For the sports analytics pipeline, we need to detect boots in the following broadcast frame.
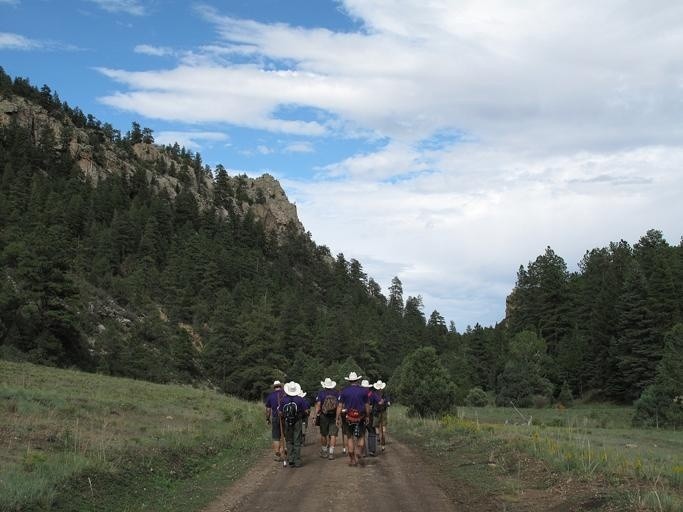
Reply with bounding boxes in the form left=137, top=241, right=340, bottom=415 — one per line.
left=355, top=445, right=367, bottom=467
left=347, top=451, right=358, bottom=466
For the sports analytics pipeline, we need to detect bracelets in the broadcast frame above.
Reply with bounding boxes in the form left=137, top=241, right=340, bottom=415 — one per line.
left=365, top=415, right=369, bottom=417
left=335, top=418, right=340, bottom=421
left=311, top=417, right=316, bottom=420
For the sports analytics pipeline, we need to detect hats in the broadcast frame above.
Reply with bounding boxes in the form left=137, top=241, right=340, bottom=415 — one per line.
left=270, top=379, right=284, bottom=390
left=373, top=380, right=387, bottom=390
left=359, top=379, right=373, bottom=388
left=297, top=390, right=307, bottom=398
left=344, top=371, right=363, bottom=382
left=283, top=381, right=301, bottom=397
left=320, top=377, right=337, bottom=389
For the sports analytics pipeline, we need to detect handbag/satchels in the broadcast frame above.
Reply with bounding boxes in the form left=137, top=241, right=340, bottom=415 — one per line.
left=345, top=406, right=363, bottom=425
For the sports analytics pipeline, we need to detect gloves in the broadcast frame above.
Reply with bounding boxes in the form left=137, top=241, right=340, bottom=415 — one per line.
left=265, top=417, right=272, bottom=427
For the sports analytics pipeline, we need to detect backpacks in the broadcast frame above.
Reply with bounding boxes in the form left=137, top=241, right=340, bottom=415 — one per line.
left=282, top=401, right=299, bottom=427
left=322, top=390, right=338, bottom=416
left=374, top=393, right=387, bottom=412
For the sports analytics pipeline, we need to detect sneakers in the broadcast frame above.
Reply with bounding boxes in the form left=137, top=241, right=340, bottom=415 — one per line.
left=319, top=450, right=328, bottom=458
left=365, top=440, right=386, bottom=457
left=327, top=452, right=336, bottom=460
left=272, top=442, right=306, bottom=470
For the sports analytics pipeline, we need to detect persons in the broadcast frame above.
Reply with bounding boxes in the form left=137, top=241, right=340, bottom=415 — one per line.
left=311, top=377, right=341, bottom=462
left=277, top=381, right=308, bottom=469
left=359, top=379, right=385, bottom=458
left=334, top=371, right=370, bottom=468
left=372, top=379, right=390, bottom=444
left=264, top=378, right=287, bottom=463
left=296, top=389, right=312, bottom=447
